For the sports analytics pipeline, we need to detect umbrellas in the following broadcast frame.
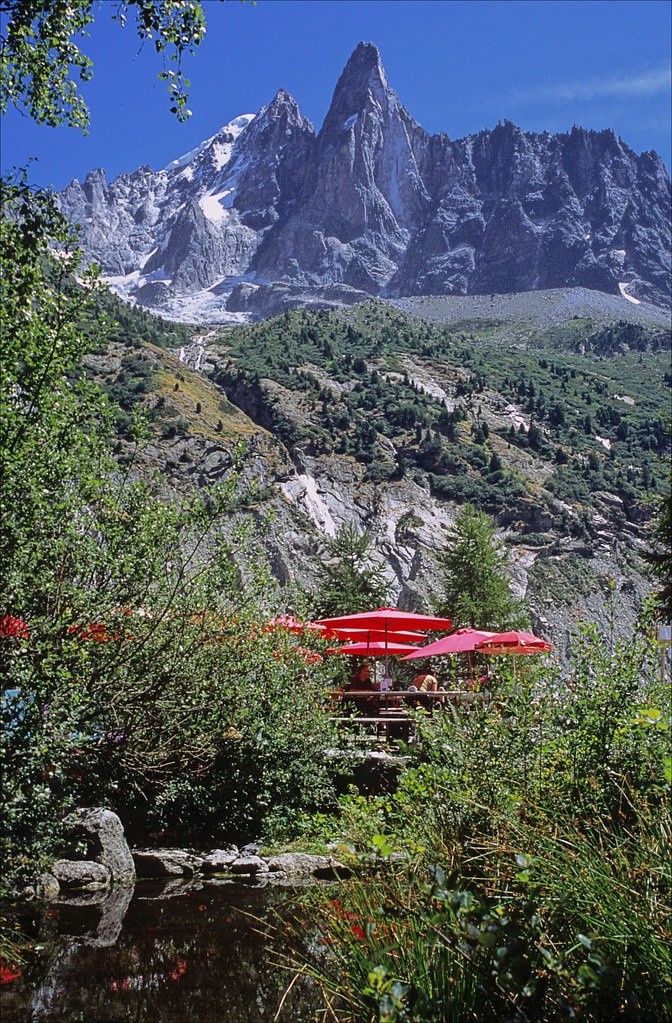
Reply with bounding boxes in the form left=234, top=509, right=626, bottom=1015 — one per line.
left=474, top=630, right=552, bottom=711
left=227, top=613, right=327, bottom=658
left=271, top=640, right=324, bottom=665
left=316, top=607, right=453, bottom=712
left=326, top=642, right=423, bottom=686
left=400, top=628, right=501, bottom=691
left=313, top=626, right=425, bottom=666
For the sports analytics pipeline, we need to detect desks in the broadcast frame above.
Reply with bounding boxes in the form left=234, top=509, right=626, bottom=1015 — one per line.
left=329, top=690, right=468, bottom=697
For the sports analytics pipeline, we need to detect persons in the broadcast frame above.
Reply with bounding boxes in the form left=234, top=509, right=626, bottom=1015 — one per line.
left=349, top=664, right=386, bottom=734
left=404, top=670, right=438, bottom=718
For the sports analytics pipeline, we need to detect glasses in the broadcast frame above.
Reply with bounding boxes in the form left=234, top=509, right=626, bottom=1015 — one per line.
left=364, top=670, right=369, bottom=672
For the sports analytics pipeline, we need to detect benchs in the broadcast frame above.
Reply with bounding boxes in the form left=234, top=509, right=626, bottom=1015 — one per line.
left=327, top=706, right=463, bottom=743
